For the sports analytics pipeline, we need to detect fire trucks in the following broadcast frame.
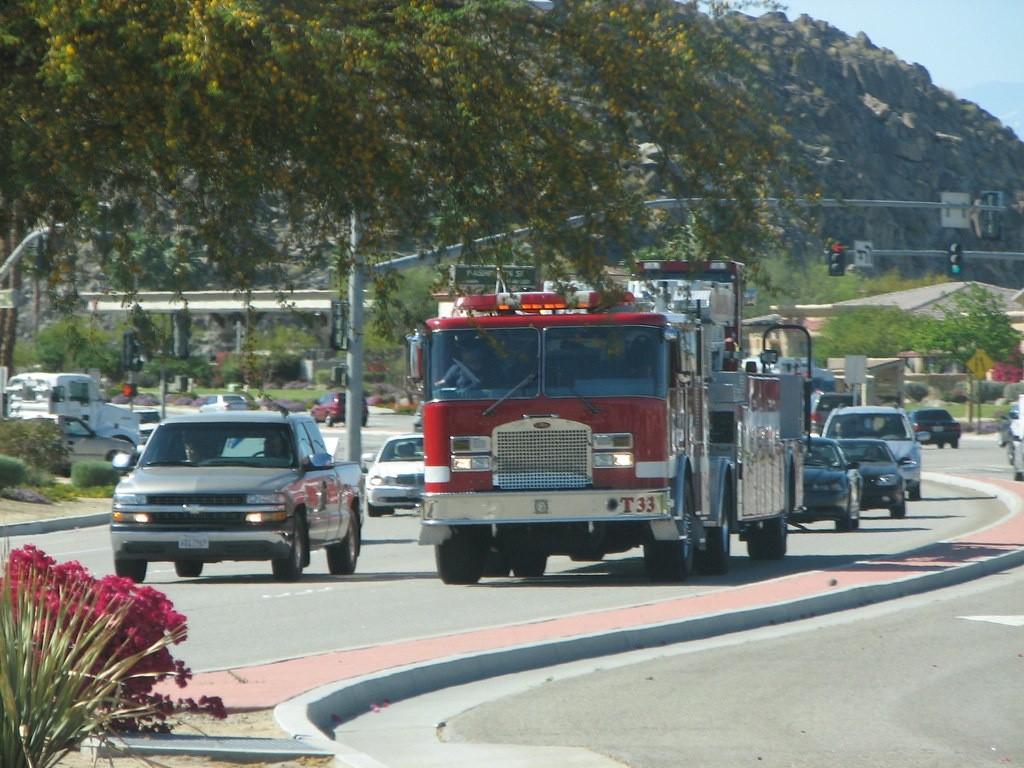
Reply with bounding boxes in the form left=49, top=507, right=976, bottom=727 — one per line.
left=406, top=259, right=813, bottom=586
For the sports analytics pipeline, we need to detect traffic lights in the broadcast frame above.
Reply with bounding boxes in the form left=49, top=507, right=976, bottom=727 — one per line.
left=945, top=239, right=964, bottom=280
left=123, top=384, right=139, bottom=398
left=829, top=240, right=845, bottom=278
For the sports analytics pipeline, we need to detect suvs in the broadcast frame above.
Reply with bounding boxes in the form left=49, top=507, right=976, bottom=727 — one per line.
left=809, top=389, right=862, bottom=439
left=822, top=402, right=930, bottom=504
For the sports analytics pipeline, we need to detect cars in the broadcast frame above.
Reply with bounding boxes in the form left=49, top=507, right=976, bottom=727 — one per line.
left=23, top=412, right=136, bottom=469
left=833, top=438, right=913, bottom=519
left=362, top=433, right=426, bottom=517
left=309, top=390, right=370, bottom=428
left=413, top=400, right=429, bottom=431
left=906, top=409, right=962, bottom=449
left=802, top=436, right=862, bottom=533
left=1000, top=393, right=1024, bottom=482
left=199, top=392, right=255, bottom=410
left=138, top=407, right=163, bottom=439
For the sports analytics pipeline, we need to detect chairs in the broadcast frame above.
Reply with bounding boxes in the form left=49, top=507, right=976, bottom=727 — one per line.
left=265, top=438, right=288, bottom=458
left=398, top=445, right=407, bottom=456
left=864, top=447, right=883, bottom=458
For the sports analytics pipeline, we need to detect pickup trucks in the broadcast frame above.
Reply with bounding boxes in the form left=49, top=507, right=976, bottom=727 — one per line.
left=110, top=410, right=365, bottom=584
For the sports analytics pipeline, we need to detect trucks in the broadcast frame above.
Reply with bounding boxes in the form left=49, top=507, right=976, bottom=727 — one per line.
left=8, top=370, right=142, bottom=451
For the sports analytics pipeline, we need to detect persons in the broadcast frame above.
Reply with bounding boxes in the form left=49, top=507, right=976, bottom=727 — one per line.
left=263, top=432, right=287, bottom=459
left=179, top=433, right=208, bottom=463
left=431, top=339, right=512, bottom=391
left=555, top=332, right=653, bottom=386
left=810, top=446, right=833, bottom=466
left=865, top=445, right=883, bottom=463
left=834, top=420, right=849, bottom=440
left=879, top=420, right=898, bottom=435
